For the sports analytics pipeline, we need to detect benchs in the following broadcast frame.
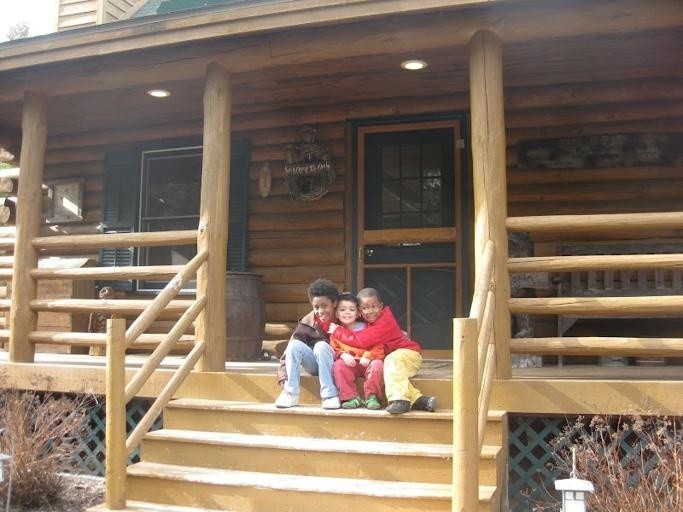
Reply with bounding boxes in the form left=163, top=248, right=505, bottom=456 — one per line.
left=550, top=236, right=683, bottom=369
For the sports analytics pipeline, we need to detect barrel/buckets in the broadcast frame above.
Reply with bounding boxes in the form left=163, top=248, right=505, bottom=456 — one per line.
left=226, top=269, right=266, bottom=360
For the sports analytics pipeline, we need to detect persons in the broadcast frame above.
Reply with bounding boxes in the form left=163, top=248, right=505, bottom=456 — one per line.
left=275, top=279, right=436, bottom=414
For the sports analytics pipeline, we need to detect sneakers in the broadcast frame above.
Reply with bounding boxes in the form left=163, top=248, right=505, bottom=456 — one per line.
left=415, top=396, right=436, bottom=412
left=275, top=390, right=299, bottom=408
left=322, top=395, right=381, bottom=410
left=386, top=400, right=410, bottom=415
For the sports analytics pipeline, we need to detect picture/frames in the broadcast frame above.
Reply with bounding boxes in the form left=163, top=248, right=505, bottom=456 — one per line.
left=46, top=175, right=85, bottom=223
